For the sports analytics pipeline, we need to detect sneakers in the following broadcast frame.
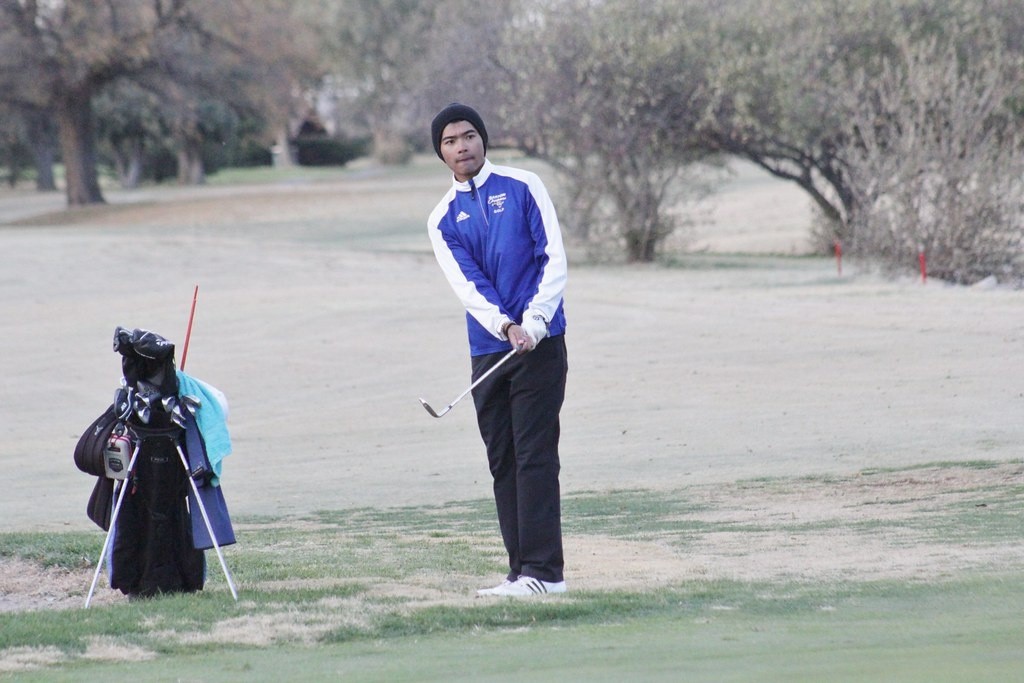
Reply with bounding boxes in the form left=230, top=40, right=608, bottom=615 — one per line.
left=473, top=573, right=566, bottom=598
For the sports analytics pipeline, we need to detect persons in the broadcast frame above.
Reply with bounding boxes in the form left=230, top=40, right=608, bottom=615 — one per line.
left=426, top=103, right=570, bottom=597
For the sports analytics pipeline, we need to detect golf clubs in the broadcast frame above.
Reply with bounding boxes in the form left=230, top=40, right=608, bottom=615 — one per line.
left=417, top=342, right=525, bottom=418
left=111, top=378, right=203, bottom=432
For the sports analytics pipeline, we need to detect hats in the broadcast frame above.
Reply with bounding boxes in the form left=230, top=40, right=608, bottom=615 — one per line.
left=430, top=101, right=488, bottom=162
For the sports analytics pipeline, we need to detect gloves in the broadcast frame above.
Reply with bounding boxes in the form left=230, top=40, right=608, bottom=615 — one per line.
left=519, top=308, right=548, bottom=350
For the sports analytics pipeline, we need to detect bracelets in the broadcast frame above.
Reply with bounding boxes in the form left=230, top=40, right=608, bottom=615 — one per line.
left=501, top=321, right=516, bottom=339
